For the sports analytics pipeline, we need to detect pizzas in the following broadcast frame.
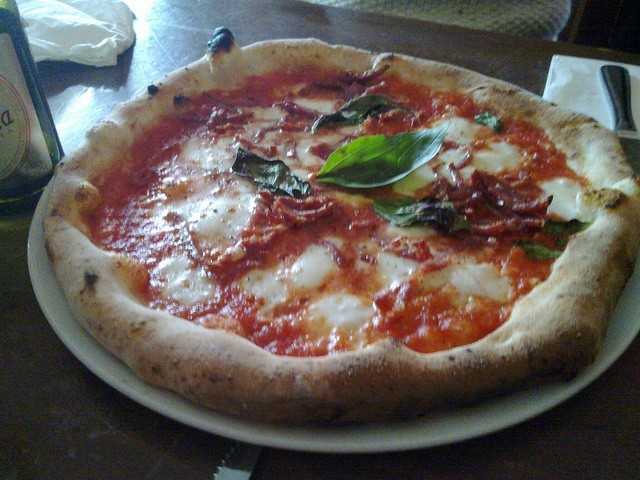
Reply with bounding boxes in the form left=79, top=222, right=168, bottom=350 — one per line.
left=43, top=28, right=640, bottom=426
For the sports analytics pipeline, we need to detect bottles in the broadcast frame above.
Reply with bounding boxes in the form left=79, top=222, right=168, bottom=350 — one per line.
left=0, top=0, right=65, bottom=212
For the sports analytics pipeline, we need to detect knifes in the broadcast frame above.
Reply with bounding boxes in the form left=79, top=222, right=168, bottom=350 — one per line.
left=602, top=59, right=640, bottom=176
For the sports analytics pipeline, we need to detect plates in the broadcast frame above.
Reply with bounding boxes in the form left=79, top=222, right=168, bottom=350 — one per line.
left=27, top=168, right=640, bottom=453
